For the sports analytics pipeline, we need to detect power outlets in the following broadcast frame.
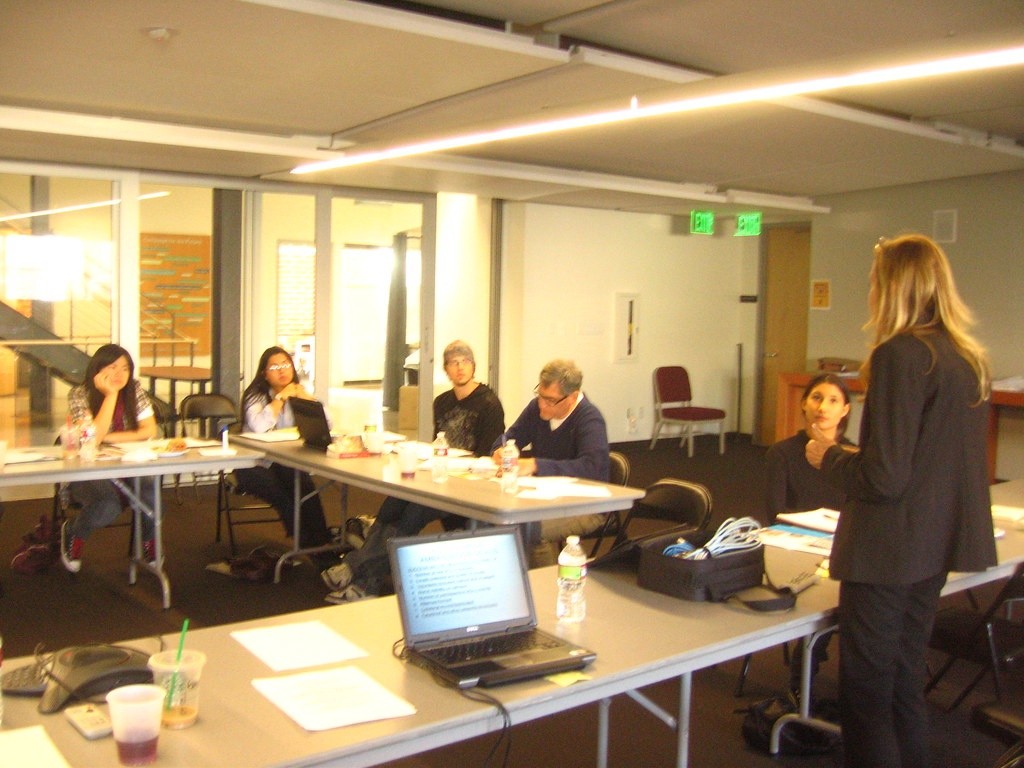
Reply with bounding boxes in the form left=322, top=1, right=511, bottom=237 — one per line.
left=626, top=408, right=632, bottom=419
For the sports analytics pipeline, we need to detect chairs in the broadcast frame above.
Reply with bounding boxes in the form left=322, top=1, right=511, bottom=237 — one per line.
left=59, top=366, right=1024, bottom=768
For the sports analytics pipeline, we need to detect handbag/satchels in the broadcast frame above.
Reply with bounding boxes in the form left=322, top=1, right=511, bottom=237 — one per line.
left=11, top=510, right=60, bottom=573
left=741, top=697, right=834, bottom=755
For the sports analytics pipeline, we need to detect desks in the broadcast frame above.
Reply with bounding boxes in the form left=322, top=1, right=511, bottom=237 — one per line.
left=0, top=484, right=1022, bottom=766
left=773, top=373, right=863, bottom=449
left=1, top=438, right=260, bottom=611
left=984, top=381, right=1024, bottom=484
left=218, top=422, right=643, bottom=588
left=139, top=366, right=211, bottom=437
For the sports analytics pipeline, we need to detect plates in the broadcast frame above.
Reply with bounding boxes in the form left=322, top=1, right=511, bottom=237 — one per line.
left=158, top=449, right=189, bottom=457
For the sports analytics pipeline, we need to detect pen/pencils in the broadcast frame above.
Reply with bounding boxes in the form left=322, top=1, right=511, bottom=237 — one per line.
left=502, top=434, right=510, bottom=450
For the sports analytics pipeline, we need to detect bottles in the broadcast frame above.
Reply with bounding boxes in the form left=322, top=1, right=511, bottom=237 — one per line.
left=557, top=534, right=587, bottom=624
left=502, top=439, right=519, bottom=492
left=80, top=415, right=96, bottom=464
left=432, top=433, right=448, bottom=482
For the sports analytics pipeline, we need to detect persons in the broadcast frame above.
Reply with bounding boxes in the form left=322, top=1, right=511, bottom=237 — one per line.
left=321, top=340, right=506, bottom=605
left=763, top=232, right=999, bottom=768
left=233, top=346, right=341, bottom=573
left=489, top=358, right=611, bottom=569
left=59, top=342, right=165, bottom=574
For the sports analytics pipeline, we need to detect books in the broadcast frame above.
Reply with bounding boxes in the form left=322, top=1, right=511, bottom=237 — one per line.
left=775, top=507, right=841, bottom=533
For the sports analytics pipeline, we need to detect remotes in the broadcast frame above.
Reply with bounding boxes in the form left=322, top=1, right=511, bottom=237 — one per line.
left=64, top=703, right=113, bottom=741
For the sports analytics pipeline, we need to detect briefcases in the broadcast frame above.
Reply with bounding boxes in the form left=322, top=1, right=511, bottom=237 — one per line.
left=588, top=522, right=797, bottom=611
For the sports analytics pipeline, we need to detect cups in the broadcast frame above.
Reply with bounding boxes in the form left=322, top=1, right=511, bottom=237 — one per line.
left=106, top=684, right=167, bottom=765
left=60, top=424, right=79, bottom=458
left=149, top=651, right=206, bottom=728
left=401, top=449, right=416, bottom=479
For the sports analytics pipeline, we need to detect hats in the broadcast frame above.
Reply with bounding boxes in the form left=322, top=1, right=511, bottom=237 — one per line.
left=443, top=340, right=473, bottom=366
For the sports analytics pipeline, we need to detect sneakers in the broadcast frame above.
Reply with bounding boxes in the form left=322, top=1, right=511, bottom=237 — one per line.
left=787, top=687, right=800, bottom=708
left=320, top=583, right=376, bottom=604
left=61, top=519, right=82, bottom=572
left=320, top=563, right=352, bottom=590
left=142, top=538, right=165, bottom=567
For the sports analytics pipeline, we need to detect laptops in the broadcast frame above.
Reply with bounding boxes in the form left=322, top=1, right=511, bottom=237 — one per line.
left=388, top=524, right=596, bottom=689
left=288, top=396, right=344, bottom=453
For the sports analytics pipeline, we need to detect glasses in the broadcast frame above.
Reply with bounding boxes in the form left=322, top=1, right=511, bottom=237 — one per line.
left=267, top=362, right=292, bottom=370
left=532, top=383, right=571, bottom=407
left=447, top=358, right=472, bottom=365
